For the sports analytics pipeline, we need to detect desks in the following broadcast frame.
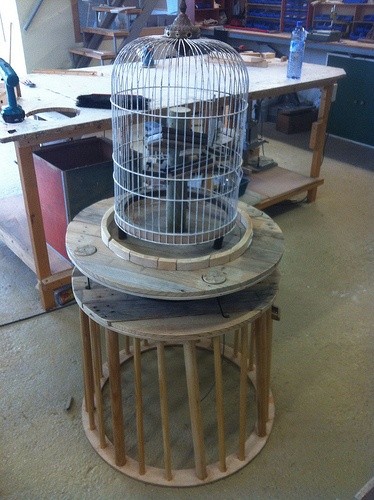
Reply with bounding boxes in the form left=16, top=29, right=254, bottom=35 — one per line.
left=0, top=55, right=347, bottom=311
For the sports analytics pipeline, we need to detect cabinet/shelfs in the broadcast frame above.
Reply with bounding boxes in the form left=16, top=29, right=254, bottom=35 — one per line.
left=325, top=54, right=374, bottom=149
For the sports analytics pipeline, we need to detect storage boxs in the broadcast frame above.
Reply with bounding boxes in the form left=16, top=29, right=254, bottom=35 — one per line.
left=33, top=135, right=144, bottom=266
left=275, top=105, right=318, bottom=135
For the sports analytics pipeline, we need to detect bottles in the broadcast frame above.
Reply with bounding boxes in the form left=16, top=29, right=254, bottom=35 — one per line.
left=287, top=21, right=308, bottom=79
